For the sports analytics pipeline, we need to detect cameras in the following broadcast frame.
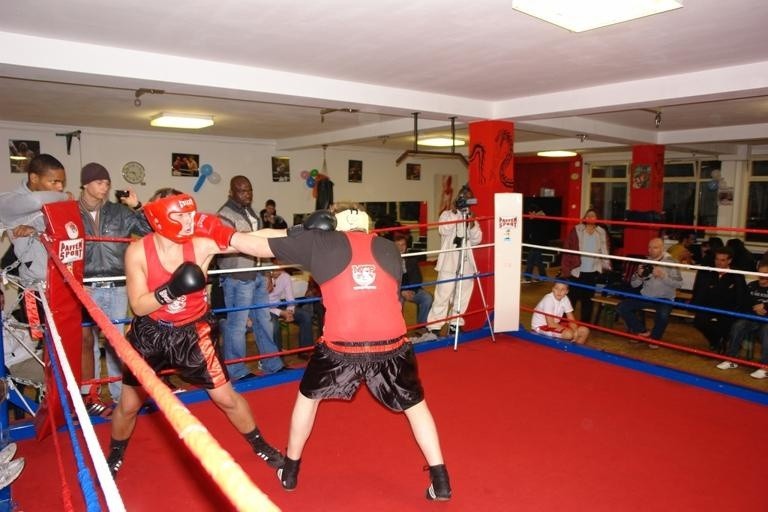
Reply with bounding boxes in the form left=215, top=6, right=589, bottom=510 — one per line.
left=453, top=194, right=479, bottom=212
left=640, top=263, right=653, bottom=276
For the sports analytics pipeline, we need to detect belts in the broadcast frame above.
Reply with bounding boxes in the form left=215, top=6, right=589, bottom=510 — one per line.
left=81, top=281, right=125, bottom=288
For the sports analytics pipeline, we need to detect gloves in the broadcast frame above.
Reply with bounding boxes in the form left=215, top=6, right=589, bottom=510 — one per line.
left=155, top=261, right=207, bottom=305
left=284, top=209, right=338, bottom=236
left=194, top=211, right=238, bottom=251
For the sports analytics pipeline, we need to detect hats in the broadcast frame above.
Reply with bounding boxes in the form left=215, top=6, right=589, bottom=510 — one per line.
left=79, top=162, right=110, bottom=190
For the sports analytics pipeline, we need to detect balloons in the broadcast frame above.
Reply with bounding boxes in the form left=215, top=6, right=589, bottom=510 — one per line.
left=718, top=179, right=728, bottom=190
left=706, top=179, right=719, bottom=191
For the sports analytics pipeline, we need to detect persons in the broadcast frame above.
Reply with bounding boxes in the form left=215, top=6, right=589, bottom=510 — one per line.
left=520, top=204, right=548, bottom=284
left=97, top=184, right=340, bottom=485
left=421, top=183, right=484, bottom=338
left=0, top=439, right=26, bottom=490
left=556, top=208, right=768, bottom=379
left=529, top=282, right=591, bottom=346
left=0, top=151, right=433, bottom=422
left=192, top=199, right=454, bottom=502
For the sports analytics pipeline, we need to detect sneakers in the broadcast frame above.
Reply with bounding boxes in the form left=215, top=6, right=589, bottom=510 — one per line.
left=107, top=449, right=124, bottom=479
left=750, top=368, right=767, bottom=379
left=252, top=437, right=285, bottom=468
left=0, top=457, right=24, bottom=490
left=424, top=483, right=451, bottom=502
left=449, top=325, right=463, bottom=336
left=716, top=360, right=738, bottom=370
left=0, top=442, right=17, bottom=464
left=275, top=465, right=298, bottom=491
left=85, top=404, right=111, bottom=417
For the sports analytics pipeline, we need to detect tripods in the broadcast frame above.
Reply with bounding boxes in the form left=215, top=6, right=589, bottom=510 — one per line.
left=439, top=221, right=497, bottom=351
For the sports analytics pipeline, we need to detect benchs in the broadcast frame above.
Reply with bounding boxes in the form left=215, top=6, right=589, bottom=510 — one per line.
left=586, top=284, right=697, bottom=333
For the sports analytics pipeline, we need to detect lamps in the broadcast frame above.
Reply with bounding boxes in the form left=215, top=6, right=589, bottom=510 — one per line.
left=149, top=112, right=215, bottom=130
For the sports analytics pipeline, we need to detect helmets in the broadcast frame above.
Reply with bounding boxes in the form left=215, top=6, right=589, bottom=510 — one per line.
left=143, top=194, right=198, bottom=246
left=331, top=208, right=370, bottom=233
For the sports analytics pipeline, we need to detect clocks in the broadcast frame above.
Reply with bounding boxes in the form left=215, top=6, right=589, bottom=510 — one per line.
left=121, top=160, right=146, bottom=185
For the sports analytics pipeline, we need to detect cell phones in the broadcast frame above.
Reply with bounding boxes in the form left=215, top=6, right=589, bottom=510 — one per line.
left=115, top=189, right=131, bottom=199
